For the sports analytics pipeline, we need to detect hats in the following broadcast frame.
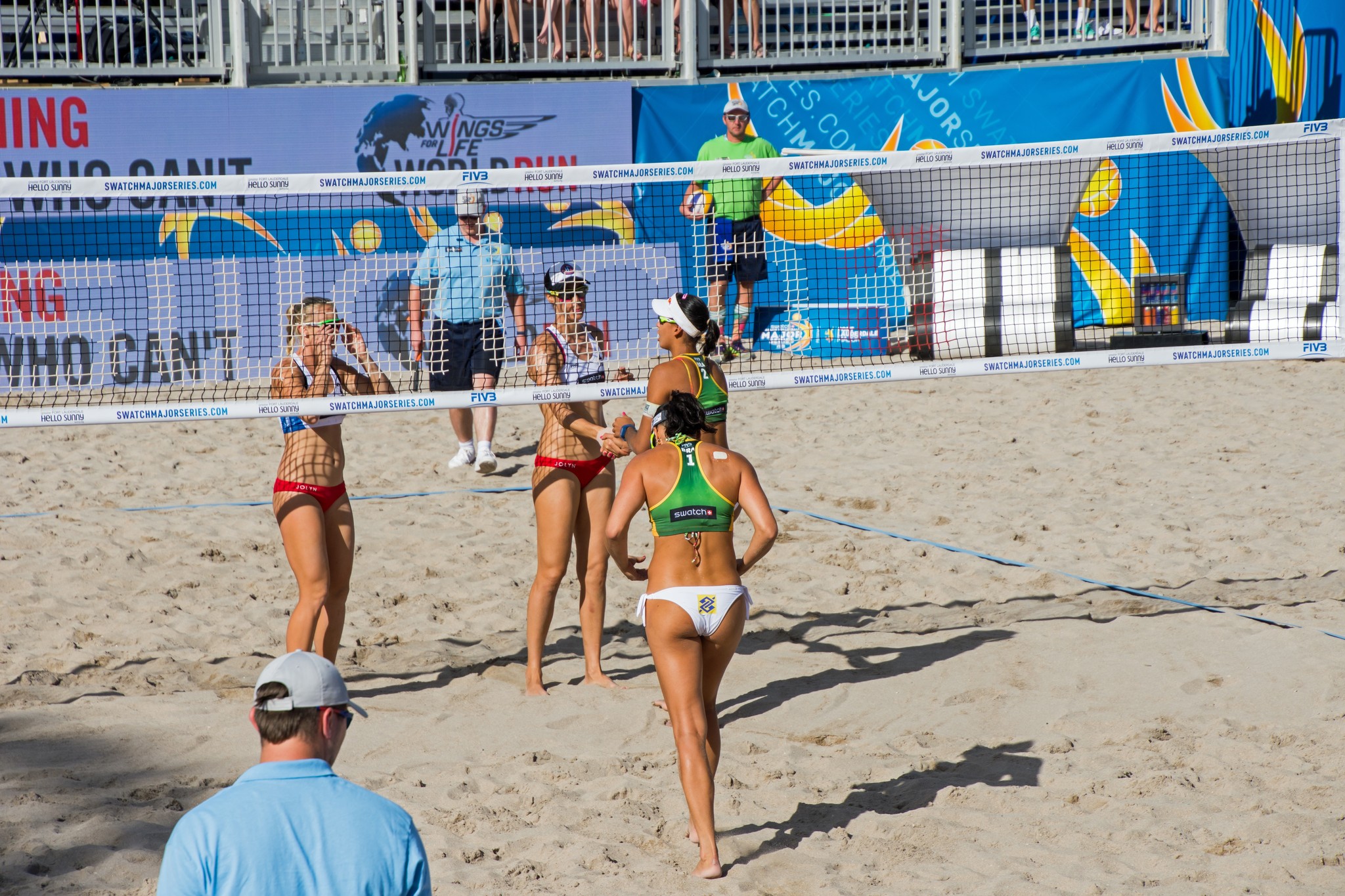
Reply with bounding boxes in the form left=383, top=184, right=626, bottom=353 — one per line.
left=455, top=189, right=484, bottom=215
left=651, top=410, right=667, bottom=449
left=652, top=292, right=707, bottom=338
left=723, top=99, right=749, bottom=113
left=252, top=649, right=368, bottom=717
left=545, top=262, right=591, bottom=291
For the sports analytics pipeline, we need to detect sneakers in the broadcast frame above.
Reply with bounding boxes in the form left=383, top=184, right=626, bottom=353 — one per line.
left=1026, top=25, right=1040, bottom=41
left=711, top=344, right=730, bottom=363
left=1074, top=21, right=1095, bottom=38
left=727, top=340, right=756, bottom=360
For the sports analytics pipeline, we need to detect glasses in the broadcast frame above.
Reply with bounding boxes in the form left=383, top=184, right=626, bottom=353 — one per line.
left=546, top=285, right=589, bottom=300
left=461, top=216, right=480, bottom=220
left=295, top=319, right=341, bottom=331
left=658, top=315, right=675, bottom=323
left=317, top=706, right=354, bottom=729
left=725, top=114, right=749, bottom=122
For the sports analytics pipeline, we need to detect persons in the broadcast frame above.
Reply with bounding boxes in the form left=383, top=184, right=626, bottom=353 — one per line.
left=478, top=0, right=681, bottom=64
left=154, top=649, right=433, bottom=896
left=600, top=292, right=730, bottom=461
left=1020, top=0, right=1096, bottom=42
left=272, top=297, right=396, bottom=664
left=604, top=390, right=779, bottom=879
left=679, top=99, right=785, bottom=364
left=522, top=262, right=631, bottom=695
left=1125, top=0, right=1164, bottom=36
left=408, top=188, right=528, bottom=474
left=710, top=0, right=769, bottom=59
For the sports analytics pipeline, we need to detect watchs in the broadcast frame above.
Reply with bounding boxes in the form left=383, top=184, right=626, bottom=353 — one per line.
left=620, top=424, right=637, bottom=441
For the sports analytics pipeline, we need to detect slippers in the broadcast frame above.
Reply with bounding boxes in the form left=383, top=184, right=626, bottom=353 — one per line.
left=623, top=48, right=640, bottom=60
left=587, top=48, right=605, bottom=59
left=753, top=44, right=769, bottom=58
left=715, top=49, right=741, bottom=58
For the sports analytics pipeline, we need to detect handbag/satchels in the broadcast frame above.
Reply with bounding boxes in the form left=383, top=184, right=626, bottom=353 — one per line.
left=474, top=34, right=513, bottom=62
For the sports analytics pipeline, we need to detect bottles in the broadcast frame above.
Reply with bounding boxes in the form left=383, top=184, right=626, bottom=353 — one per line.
left=397, top=50, right=405, bottom=82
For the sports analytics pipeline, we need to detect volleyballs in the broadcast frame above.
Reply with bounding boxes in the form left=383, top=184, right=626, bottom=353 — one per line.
left=687, top=188, right=716, bottom=218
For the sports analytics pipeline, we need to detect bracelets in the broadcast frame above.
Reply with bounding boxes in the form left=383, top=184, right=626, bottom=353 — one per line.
left=516, top=331, right=526, bottom=335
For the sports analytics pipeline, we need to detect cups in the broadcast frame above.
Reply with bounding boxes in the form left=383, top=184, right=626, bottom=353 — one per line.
left=705, top=69, right=720, bottom=77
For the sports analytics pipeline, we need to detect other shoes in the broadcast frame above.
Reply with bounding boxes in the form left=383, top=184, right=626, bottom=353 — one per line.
left=475, top=450, right=497, bottom=474
left=511, top=42, right=526, bottom=59
left=1090, top=20, right=1104, bottom=37
left=448, top=448, right=476, bottom=468
left=1102, top=19, right=1123, bottom=35
